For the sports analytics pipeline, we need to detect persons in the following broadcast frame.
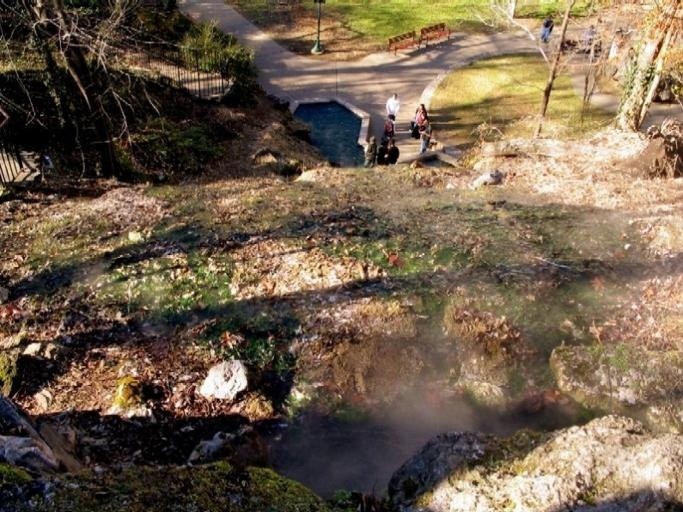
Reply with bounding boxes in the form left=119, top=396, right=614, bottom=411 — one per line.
left=541, top=14, right=553, bottom=41
left=584, top=19, right=634, bottom=82
left=365, top=93, right=431, bottom=169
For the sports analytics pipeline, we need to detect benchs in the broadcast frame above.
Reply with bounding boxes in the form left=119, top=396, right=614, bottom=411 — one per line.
left=386, top=22, right=452, bottom=56
